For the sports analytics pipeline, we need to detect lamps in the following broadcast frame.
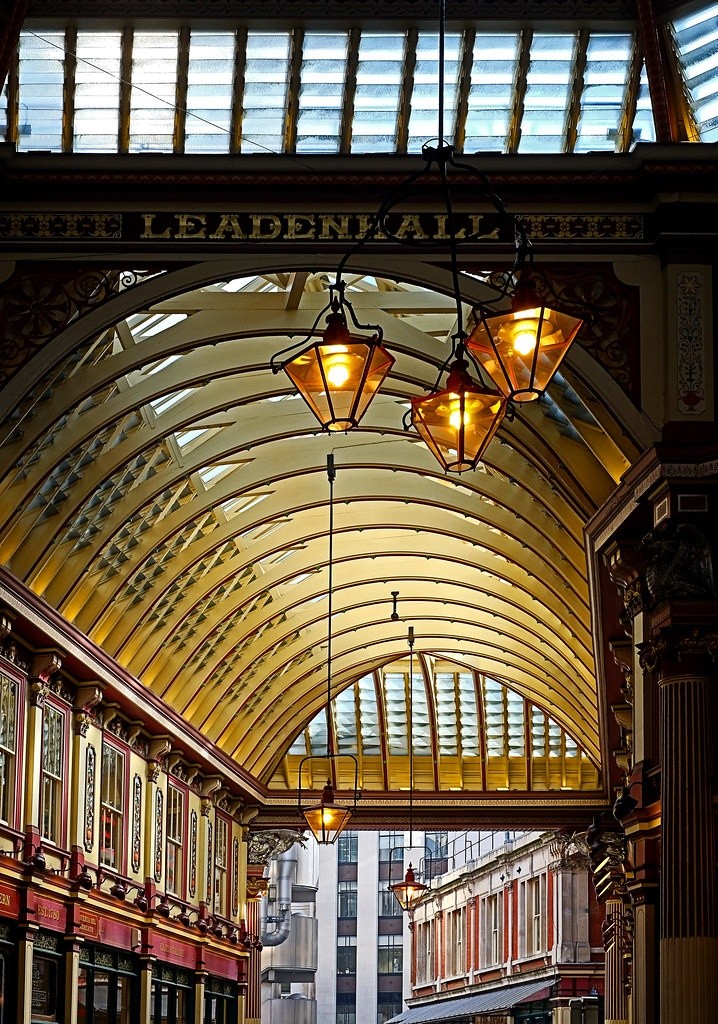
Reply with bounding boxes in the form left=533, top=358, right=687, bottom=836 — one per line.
left=388, top=626, right=432, bottom=911
left=208, top=924, right=238, bottom=944
left=0, top=843, right=46, bottom=873
left=612, top=782, right=660, bottom=820
left=269, top=0, right=595, bottom=475
left=169, top=904, right=189, bottom=928
left=299, top=454, right=360, bottom=844
left=248, top=934, right=263, bottom=952
left=125, top=887, right=148, bottom=914
left=588, top=829, right=625, bottom=862
left=586, top=810, right=611, bottom=846
left=91, top=875, right=125, bottom=901
left=223, top=929, right=251, bottom=948
left=191, top=916, right=222, bottom=938
left=149, top=895, right=170, bottom=919
left=51, top=863, right=92, bottom=890
left=173, top=910, right=208, bottom=934
